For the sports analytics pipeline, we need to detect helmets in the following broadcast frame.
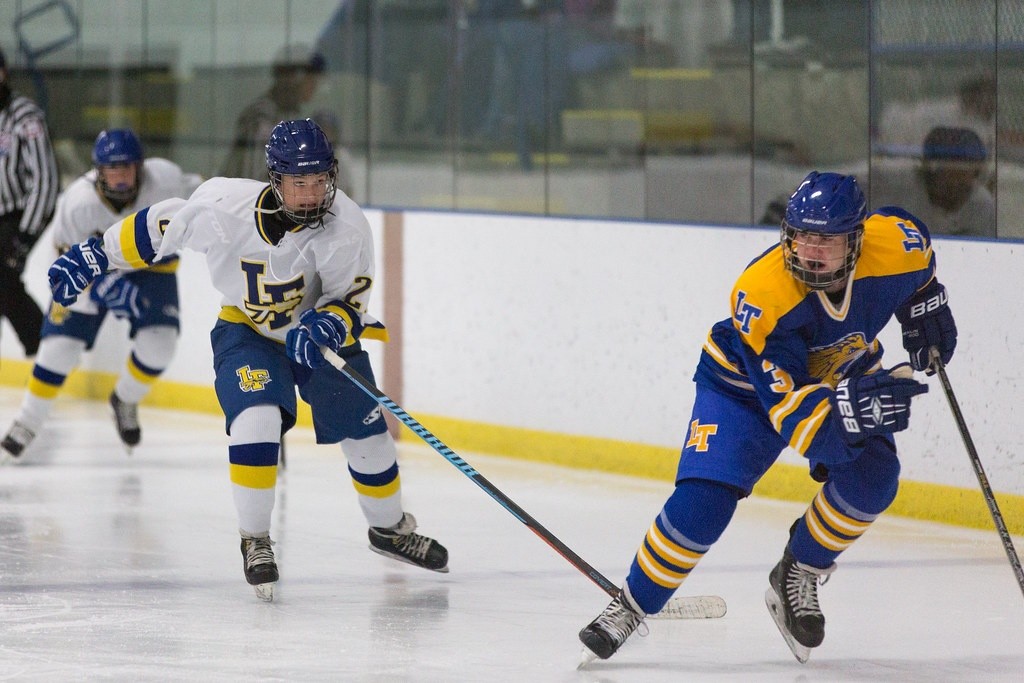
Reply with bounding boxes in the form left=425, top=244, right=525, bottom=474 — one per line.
left=779, top=172, right=868, bottom=290
left=921, top=124, right=987, bottom=205
left=91, top=127, right=144, bottom=201
left=264, top=118, right=340, bottom=226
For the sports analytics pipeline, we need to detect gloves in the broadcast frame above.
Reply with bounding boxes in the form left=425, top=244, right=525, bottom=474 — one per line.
left=90, top=270, right=152, bottom=321
left=6, top=233, right=36, bottom=269
left=48, top=236, right=108, bottom=308
left=834, top=363, right=928, bottom=445
left=286, top=302, right=361, bottom=369
left=894, top=277, right=957, bottom=376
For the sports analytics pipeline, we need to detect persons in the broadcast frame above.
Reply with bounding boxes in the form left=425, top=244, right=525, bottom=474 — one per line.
left=578, top=168, right=959, bottom=660
left=758, top=122, right=995, bottom=238
left=0, top=46, right=59, bottom=356
left=219, top=40, right=328, bottom=181
left=942, top=77, right=993, bottom=150
left=48, top=119, right=449, bottom=584
left=1, top=127, right=206, bottom=459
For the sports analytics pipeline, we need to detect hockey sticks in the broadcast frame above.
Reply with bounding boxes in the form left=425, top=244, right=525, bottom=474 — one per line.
left=296, top=326, right=728, bottom=621
left=933, top=349, right=1024, bottom=594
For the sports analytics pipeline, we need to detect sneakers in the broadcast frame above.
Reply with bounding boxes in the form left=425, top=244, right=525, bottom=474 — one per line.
left=240, top=530, right=279, bottom=602
left=577, top=581, right=649, bottom=668
left=0, top=421, right=35, bottom=461
left=763, top=517, right=838, bottom=664
left=108, top=389, right=140, bottom=453
left=367, top=512, right=449, bottom=573
